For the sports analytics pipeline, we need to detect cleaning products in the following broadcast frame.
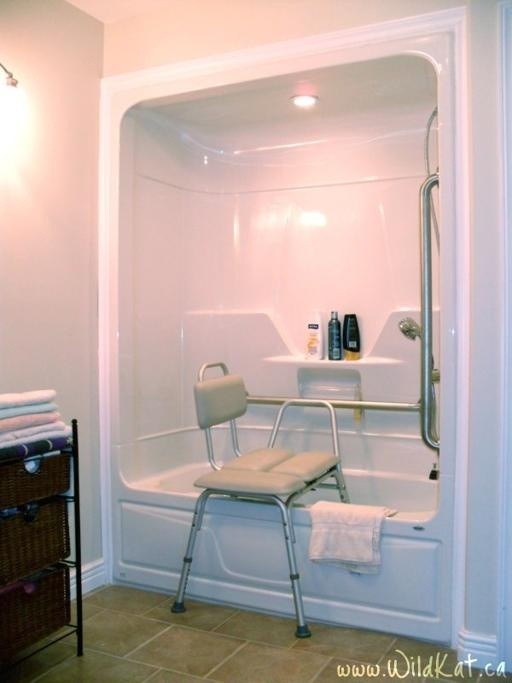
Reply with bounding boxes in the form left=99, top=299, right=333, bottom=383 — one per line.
left=328, top=312, right=341, bottom=361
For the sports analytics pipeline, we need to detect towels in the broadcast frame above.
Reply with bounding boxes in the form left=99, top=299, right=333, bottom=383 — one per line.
left=0, top=388, right=78, bottom=446
left=310, top=500, right=395, bottom=573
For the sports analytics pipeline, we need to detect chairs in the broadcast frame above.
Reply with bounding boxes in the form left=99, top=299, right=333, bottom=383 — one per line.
left=171, top=362, right=353, bottom=638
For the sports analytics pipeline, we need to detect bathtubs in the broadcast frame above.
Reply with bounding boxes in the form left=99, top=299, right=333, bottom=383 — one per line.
left=125, top=462, right=437, bottom=525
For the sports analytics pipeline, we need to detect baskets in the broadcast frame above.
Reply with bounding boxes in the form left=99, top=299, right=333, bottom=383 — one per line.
left=0, top=455, right=74, bottom=513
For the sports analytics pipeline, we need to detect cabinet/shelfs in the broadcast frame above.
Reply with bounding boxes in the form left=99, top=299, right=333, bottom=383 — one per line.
left=0, top=419, right=84, bottom=681
